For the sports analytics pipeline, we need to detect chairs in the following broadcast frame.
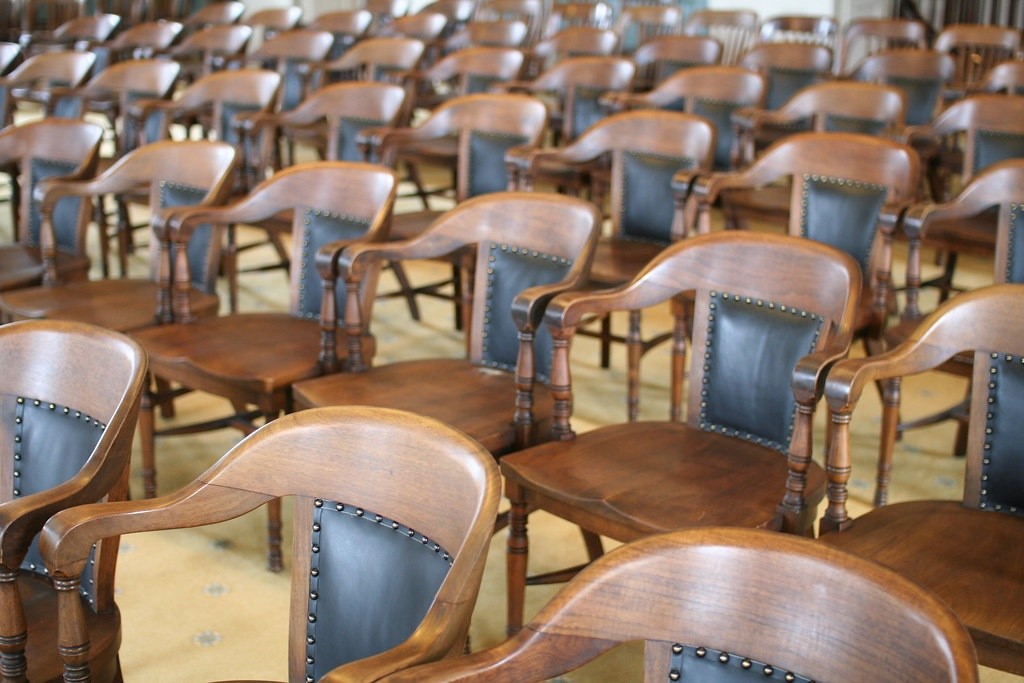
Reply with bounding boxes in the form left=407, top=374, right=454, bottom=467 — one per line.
left=1, top=1, right=1023, bottom=683
